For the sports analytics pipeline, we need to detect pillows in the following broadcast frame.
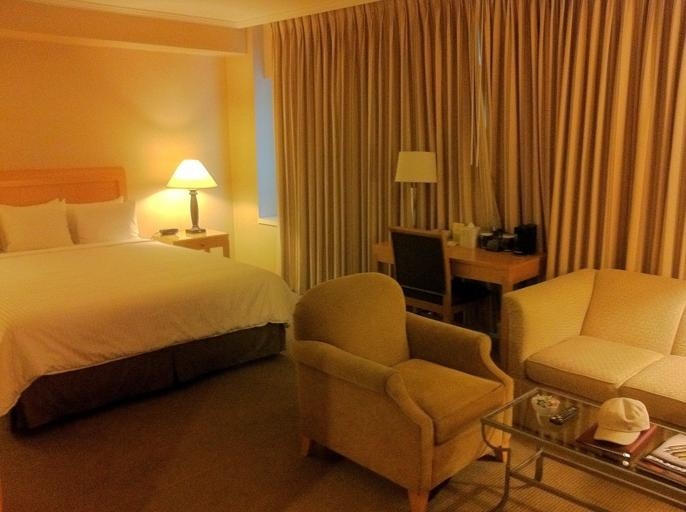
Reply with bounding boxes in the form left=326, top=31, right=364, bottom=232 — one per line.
left=1, top=196, right=138, bottom=254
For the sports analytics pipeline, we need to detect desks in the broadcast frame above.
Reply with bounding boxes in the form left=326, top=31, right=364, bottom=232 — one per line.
left=370, top=237, right=548, bottom=328
left=151, top=226, right=231, bottom=259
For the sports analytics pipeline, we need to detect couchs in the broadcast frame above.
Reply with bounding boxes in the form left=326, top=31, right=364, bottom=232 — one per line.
left=498, top=264, right=685, bottom=453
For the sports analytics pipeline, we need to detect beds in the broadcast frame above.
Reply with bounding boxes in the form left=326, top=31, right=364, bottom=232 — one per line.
left=0, top=163, right=298, bottom=438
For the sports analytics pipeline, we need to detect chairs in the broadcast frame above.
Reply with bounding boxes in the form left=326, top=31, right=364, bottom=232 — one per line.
left=388, top=227, right=498, bottom=337
left=284, top=268, right=515, bottom=511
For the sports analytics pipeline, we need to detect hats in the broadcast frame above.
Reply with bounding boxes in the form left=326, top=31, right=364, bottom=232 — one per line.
left=591, top=396, right=652, bottom=446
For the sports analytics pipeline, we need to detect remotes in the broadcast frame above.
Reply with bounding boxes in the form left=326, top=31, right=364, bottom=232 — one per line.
left=550, top=405, right=579, bottom=425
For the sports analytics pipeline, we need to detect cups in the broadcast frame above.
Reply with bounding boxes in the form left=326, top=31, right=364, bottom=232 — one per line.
left=451, top=222, right=465, bottom=245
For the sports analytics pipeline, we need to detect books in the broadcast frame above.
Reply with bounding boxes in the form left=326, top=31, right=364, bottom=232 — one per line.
left=576, top=418, right=656, bottom=457
left=635, top=433, right=686, bottom=489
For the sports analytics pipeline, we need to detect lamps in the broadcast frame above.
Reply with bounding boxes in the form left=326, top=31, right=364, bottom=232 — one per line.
left=392, top=151, right=439, bottom=226
left=164, top=158, right=220, bottom=235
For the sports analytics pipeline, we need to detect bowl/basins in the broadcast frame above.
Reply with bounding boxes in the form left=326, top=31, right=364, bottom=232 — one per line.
left=531, top=397, right=560, bottom=416
